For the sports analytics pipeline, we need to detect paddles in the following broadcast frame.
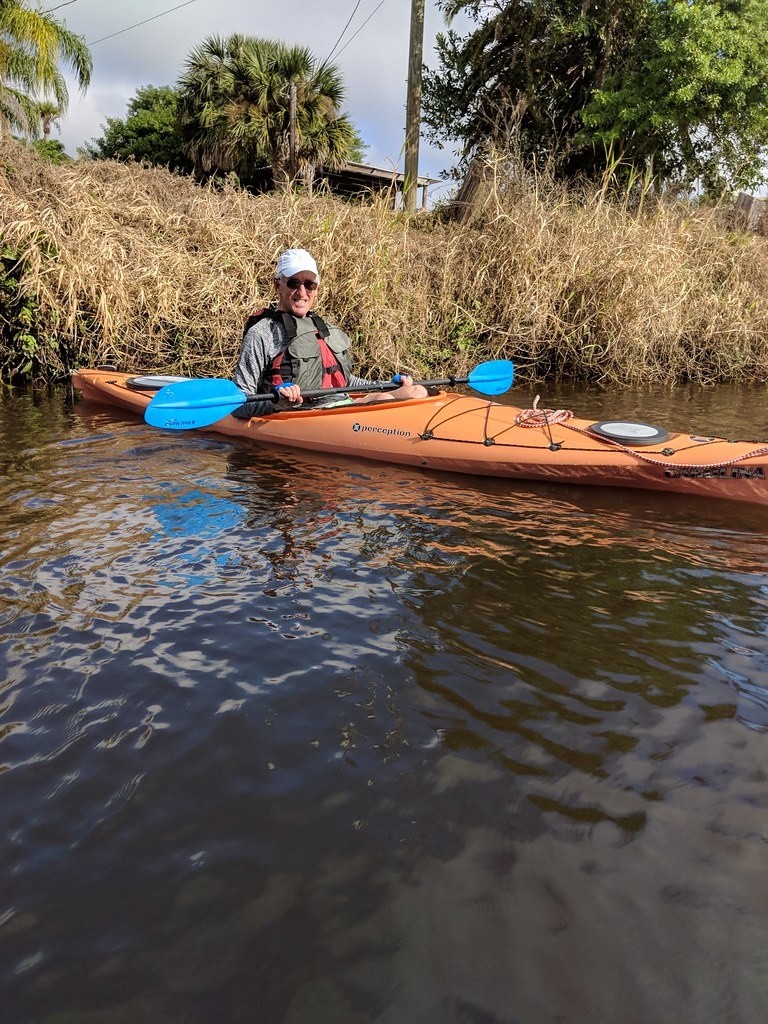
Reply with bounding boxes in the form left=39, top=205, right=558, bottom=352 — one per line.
left=143, top=358, right=519, bottom=431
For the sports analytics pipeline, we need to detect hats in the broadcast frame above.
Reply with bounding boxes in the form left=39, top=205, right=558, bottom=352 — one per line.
left=274, top=249, right=318, bottom=279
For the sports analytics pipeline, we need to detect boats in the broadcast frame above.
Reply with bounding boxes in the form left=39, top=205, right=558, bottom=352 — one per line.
left=71, top=367, right=768, bottom=506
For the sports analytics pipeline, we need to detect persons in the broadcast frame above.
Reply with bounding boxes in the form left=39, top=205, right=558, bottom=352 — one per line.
left=229, top=249, right=432, bottom=419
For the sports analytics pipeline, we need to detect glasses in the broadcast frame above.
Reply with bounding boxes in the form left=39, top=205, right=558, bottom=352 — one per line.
left=282, top=278, right=317, bottom=290
